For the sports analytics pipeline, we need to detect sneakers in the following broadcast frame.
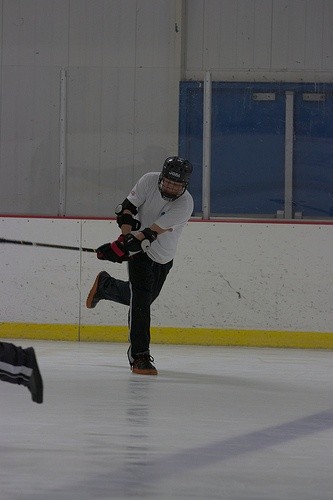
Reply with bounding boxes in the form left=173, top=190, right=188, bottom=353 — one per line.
left=25, top=347, right=44, bottom=404
left=130, top=354, right=157, bottom=375
left=86, top=271, right=110, bottom=308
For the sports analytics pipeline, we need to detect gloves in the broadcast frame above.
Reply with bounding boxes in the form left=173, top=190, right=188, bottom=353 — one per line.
left=96, top=241, right=127, bottom=262
left=109, top=234, right=130, bottom=263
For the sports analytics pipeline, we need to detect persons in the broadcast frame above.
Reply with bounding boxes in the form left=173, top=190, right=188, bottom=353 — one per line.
left=0, top=341, right=43, bottom=403
left=85, top=157, right=195, bottom=378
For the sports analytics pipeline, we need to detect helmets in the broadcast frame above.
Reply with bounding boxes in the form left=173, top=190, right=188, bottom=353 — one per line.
left=157, top=156, right=193, bottom=202
